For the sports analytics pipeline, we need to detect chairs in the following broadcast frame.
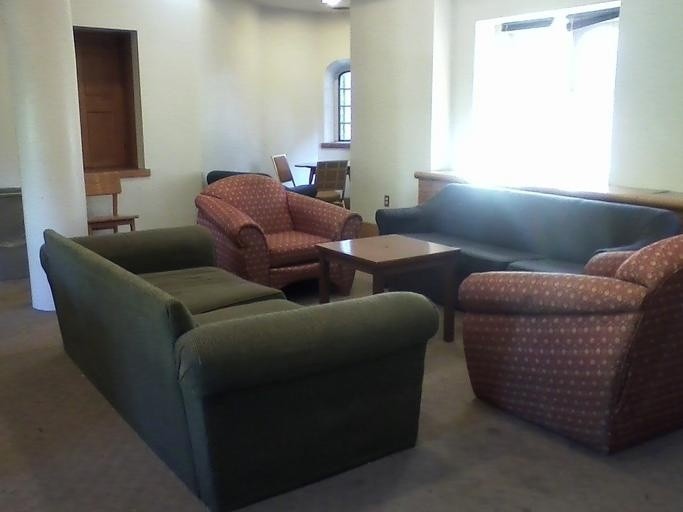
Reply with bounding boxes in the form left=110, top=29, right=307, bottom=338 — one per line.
left=456, top=234, right=682, bottom=455
left=193, top=173, right=363, bottom=297
left=83, top=170, right=139, bottom=237
left=206, top=153, right=350, bottom=210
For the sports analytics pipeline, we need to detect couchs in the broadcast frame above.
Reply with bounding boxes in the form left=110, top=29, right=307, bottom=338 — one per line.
left=39, top=226, right=438, bottom=511
left=375, top=182, right=677, bottom=313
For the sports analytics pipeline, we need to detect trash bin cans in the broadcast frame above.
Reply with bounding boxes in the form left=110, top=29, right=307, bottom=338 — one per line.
left=0, top=187, right=30, bottom=280
